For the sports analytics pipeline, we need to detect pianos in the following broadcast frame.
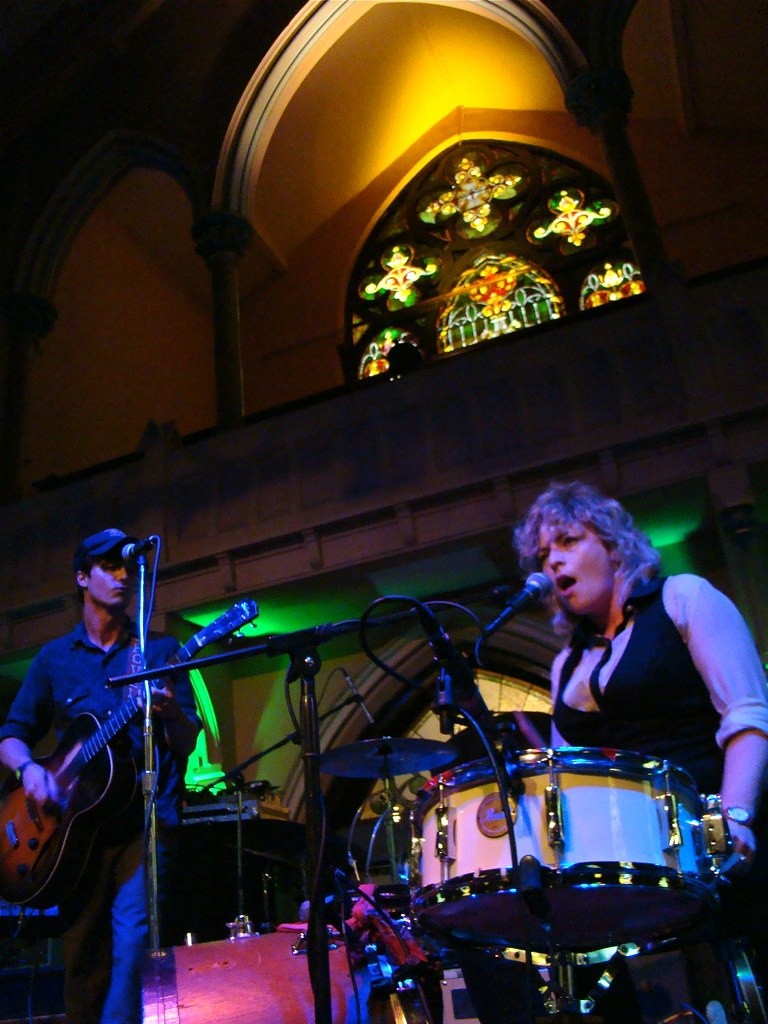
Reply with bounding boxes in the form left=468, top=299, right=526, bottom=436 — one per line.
left=179, top=800, right=298, bottom=827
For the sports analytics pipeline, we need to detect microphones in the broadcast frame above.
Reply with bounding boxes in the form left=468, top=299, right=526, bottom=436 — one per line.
left=342, top=668, right=376, bottom=724
left=121, top=535, right=157, bottom=564
left=483, top=573, right=554, bottom=640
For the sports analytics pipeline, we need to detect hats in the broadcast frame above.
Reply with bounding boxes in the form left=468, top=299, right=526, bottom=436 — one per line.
left=74, top=528, right=139, bottom=571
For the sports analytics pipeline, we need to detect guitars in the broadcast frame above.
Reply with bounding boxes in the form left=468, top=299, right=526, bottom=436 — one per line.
left=0, top=597, right=260, bottom=911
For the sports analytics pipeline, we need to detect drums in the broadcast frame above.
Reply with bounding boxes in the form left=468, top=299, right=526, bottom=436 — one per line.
left=141, top=926, right=364, bottom=1024
left=413, top=744, right=724, bottom=966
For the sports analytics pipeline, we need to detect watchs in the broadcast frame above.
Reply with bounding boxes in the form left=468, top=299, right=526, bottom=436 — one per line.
left=16, top=759, right=34, bottom=781
left=723, top=806, right=756, bottom=830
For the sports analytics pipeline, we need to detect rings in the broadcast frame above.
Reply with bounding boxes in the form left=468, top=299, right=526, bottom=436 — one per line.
left=733, top=851, right=747, bottom=861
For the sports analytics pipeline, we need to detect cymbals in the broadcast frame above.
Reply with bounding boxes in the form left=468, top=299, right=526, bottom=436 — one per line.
left=318, top=731, right=460, bottom=779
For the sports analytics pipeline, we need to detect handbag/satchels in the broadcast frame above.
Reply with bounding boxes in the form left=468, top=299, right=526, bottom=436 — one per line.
left=141, top=922, right=371, bottom=1024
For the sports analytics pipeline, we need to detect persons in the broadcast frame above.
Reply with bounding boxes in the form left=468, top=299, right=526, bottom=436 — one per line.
left=0, top=530, right=201, bottom=1024
left=512, top=484, right=768, bottom=882
